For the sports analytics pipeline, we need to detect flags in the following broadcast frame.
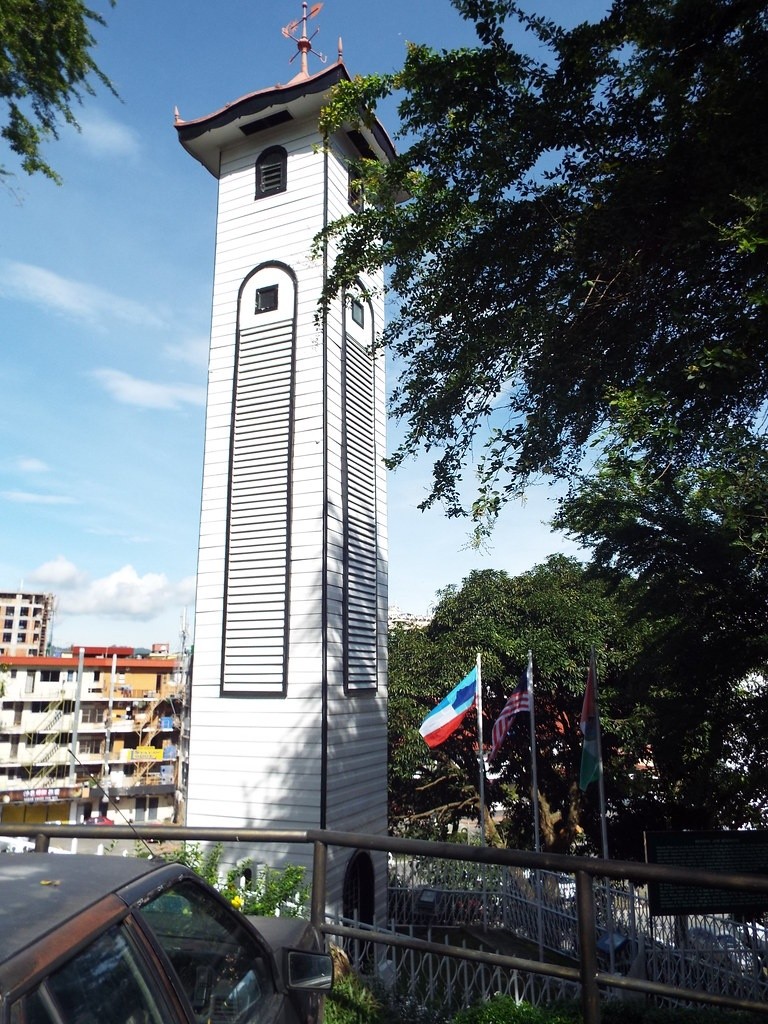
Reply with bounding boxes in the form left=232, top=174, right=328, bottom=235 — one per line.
left=485, top=658, right=533, bottom=762
left=579, top=654, right=599, bottom=792
left=418, top=662, right=482, bottom=749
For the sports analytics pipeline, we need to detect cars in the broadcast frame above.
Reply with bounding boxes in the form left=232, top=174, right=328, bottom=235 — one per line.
left=0, top=851, right=335, bottom=1024
left=520, top=867, right=578, bottom=907
left=81, top=816, right=116, bottom=828
left=686, top=925, right=763, bottom=975
